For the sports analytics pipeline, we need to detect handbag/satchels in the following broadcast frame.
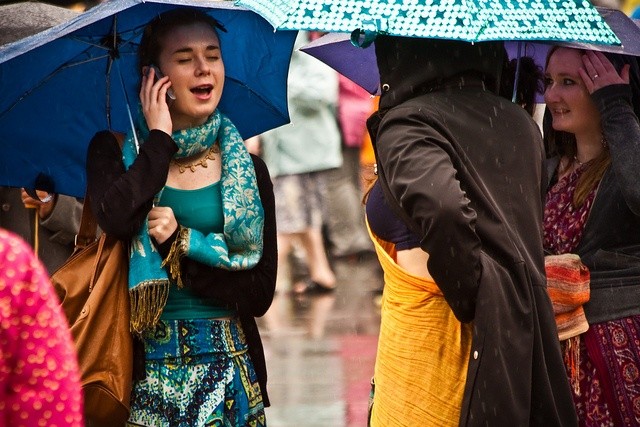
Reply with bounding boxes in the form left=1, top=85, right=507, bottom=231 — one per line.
left=51, top=130, right=134, bottom=427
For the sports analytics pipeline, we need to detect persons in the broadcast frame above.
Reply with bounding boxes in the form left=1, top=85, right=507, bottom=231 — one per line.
left=536, top=42, right=640, bottom=426
left=362, top=31, right=579, bottom=425
left=253, top=30, right=344, bottom=295
left=83, top=8, right=278, bottom=425
left=0, top=184, right=103, bottom=278
left=0, top=226, right=84, bottom=425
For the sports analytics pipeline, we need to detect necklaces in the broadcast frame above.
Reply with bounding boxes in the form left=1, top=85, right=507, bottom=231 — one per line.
left=169, top=140, right=221, bottom=173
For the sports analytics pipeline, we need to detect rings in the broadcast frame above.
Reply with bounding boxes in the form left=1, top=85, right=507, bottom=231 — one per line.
left=591, top=74, right=599, bottom=81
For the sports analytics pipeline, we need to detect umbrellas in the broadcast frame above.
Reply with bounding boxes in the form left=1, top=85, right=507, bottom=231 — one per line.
left=0, top=0, right=299, bottom=207
left=229, top=0, right=625, bottom=49
left=0, top=2, right=82, bottom=46
left=299, top=6, right=640, bottom=104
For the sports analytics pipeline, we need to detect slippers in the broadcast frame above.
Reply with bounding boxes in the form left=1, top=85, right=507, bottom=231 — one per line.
left=291, top=279, right=336, bottom=296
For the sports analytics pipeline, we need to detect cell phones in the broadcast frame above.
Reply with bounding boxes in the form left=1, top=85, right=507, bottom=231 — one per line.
left=144, top=64, right=176, bottom=108
